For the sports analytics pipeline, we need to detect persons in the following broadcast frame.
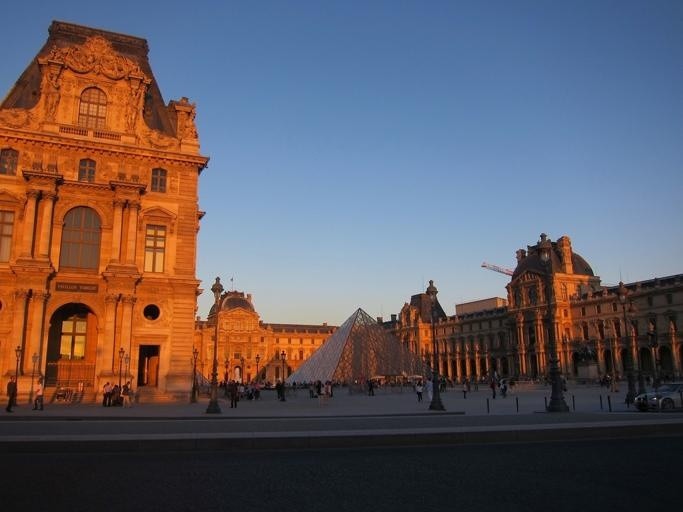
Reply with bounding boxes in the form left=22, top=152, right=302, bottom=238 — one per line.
left=218, top=378, right=334, bottom=409
left=645, top=372, right=676, bottom=387
left=6, top=376, right=18, bottom=412
left=102, top=381, right=132, bottom=409
left=367, top=375, right=507, bottom=402
left=31, top=375, right=43, bottom=411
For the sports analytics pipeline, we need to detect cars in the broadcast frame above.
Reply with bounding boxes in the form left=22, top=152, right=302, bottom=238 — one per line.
left=632, top=382, right=682, bottom=412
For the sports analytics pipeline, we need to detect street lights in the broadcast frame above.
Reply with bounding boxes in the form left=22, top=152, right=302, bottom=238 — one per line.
left=425, top=279, right=445, bottom=411
left=14, top=346, right=22, bottom=406
left=615, top=282, right=638, bottom=404
left=240, top=357, right=244, bottom=384
left=255, top=354, right=259, bottom=375
left=280, top=350, right=286, bottom=401
left=536, top=232, right=570, bottom=412
left=118, top=347, right=124, bottom=389
left=191, top=349, right=198, bottom=403
left=206, top=276, right=224, bottom=414
left=224, top=358, right=229, bottom=384
